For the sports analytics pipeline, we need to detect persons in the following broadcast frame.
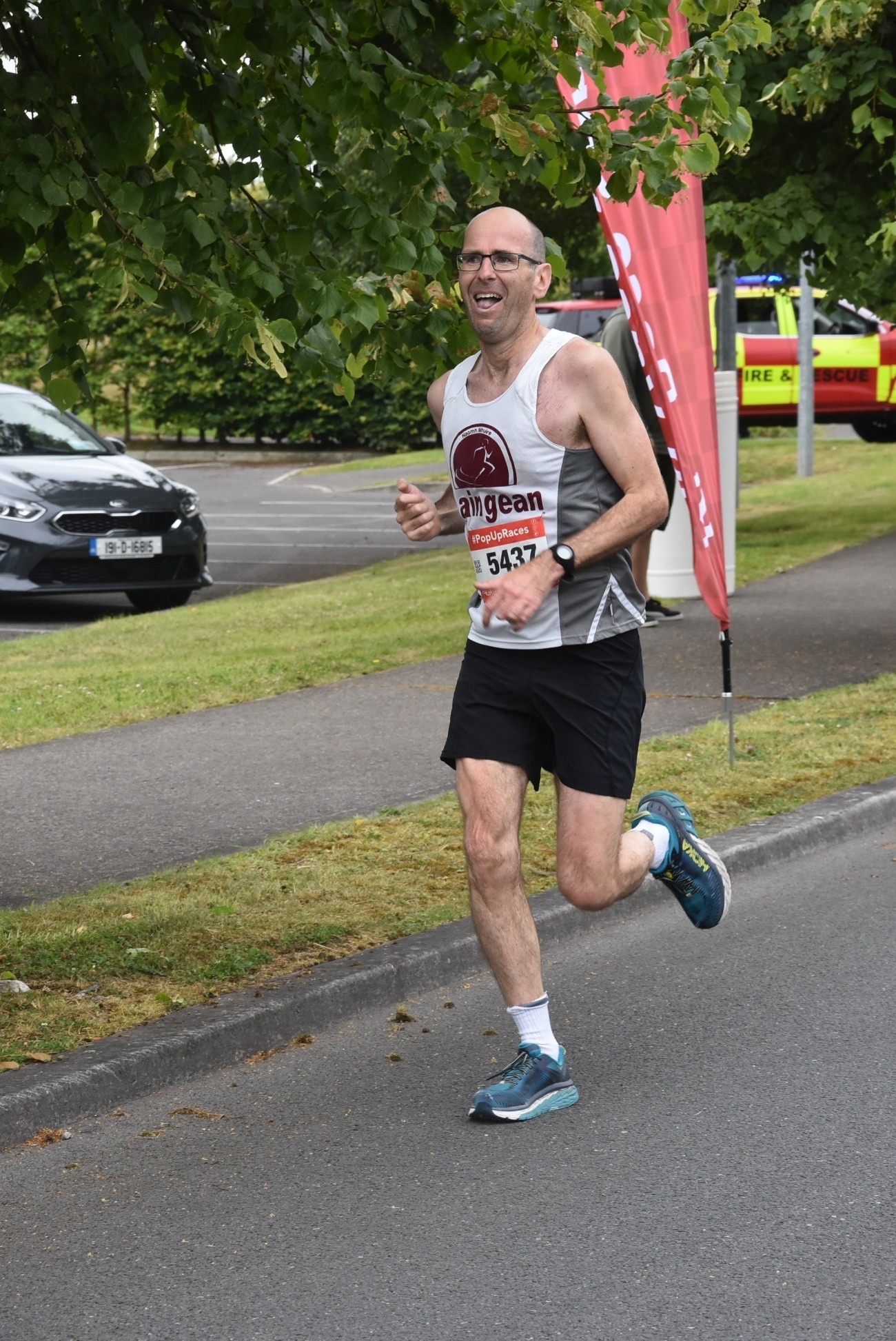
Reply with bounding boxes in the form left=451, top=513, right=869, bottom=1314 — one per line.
left=392, top=205, right=732, bottom=1126
left=599, top=304, right=684, bottom=630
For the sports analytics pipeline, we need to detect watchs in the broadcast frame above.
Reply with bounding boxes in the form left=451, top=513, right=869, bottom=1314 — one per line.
left=548, top=541, right=576, bottom=582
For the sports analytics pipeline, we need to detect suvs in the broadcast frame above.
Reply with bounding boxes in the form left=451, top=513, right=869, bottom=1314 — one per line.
left=533, top=278, right=624, bottom=341
left=583, top=272, right=896, bottom=448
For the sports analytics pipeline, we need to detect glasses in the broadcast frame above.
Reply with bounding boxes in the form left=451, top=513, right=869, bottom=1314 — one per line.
left=456, top=252, right=542, bottom=271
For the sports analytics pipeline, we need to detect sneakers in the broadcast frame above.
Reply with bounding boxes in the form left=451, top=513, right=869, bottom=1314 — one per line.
left=644, top=598, right=683, bottom=622
left=467, top=1043, right=579, bottom=1121
left=632, top=790, right=731, bottom=930
left=639, top=615, right=659, bottom=629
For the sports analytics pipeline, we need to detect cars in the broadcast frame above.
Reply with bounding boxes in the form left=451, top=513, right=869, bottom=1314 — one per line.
left=0, top=380, right=216, bottom=611
